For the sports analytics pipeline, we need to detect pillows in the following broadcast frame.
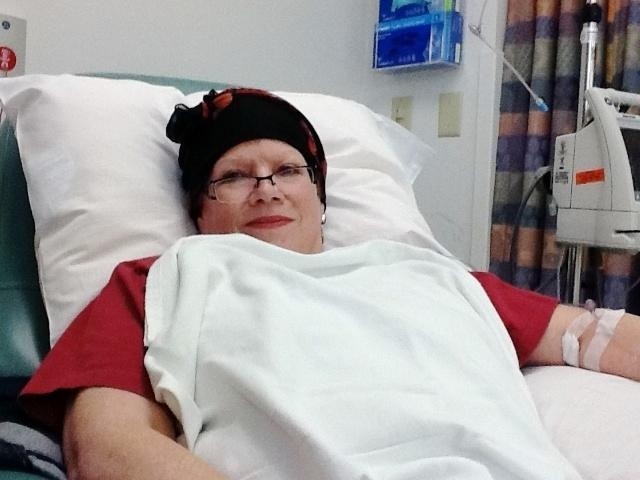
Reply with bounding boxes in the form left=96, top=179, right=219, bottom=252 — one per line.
left=2, top=73, right=438, bottom=348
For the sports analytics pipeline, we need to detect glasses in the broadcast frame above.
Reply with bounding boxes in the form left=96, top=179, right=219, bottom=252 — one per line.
left=209, top=165, right=320, bottom=207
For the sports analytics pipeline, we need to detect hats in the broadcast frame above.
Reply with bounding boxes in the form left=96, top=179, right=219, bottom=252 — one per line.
left=165, top=88, right=332, bottom=194
